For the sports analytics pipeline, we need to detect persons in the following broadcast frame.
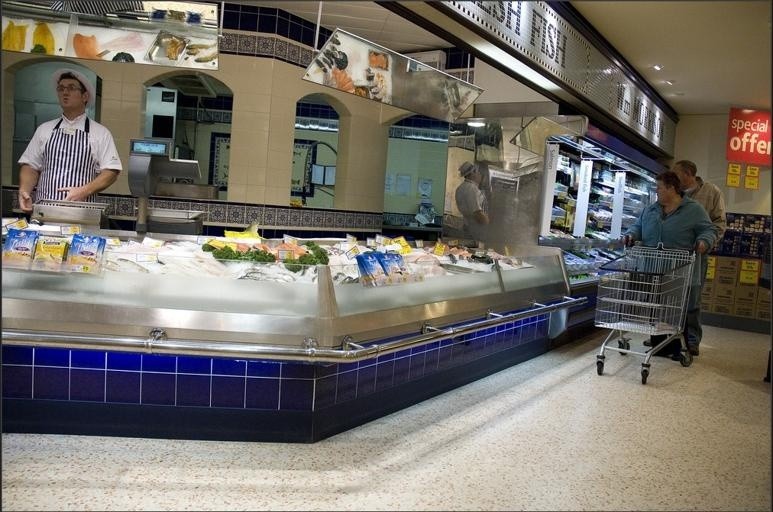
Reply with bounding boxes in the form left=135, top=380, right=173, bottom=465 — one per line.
left=621, top=160, right=727, bottom=361
left=18, top=68, right=123, bottom=215
left=455, top=161, right=490, bottom=240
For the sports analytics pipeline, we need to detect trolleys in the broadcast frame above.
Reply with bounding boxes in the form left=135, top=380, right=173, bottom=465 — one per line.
left=593, top=241, right=696, bottom=385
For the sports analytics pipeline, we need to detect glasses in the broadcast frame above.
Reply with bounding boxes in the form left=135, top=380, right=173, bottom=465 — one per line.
left=55, top=84, right=83, bottom=93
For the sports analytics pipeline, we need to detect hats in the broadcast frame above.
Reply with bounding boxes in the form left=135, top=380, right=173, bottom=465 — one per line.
left=51, top=69, right=95, bottom=106
left=456, top=160, right=479, bottom=178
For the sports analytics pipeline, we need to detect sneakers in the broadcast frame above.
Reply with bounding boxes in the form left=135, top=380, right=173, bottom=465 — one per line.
left=641, top=337, right=701, bottom=361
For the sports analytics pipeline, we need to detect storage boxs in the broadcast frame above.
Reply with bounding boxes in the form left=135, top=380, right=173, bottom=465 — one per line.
left=699, top=254, right=770, bottom=322
